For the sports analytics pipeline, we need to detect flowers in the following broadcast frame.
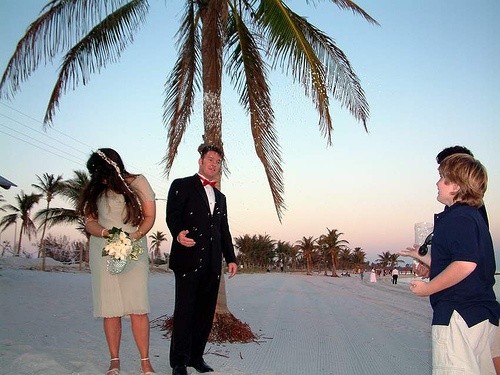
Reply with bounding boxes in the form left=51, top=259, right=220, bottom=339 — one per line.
left=102, top=226, right=143, bottom=261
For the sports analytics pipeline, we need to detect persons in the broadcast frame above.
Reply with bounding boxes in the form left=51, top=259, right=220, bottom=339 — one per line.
left=166, top=145, right=238, bottom=375
left=79, top=148, right=156, bottom=375
left=395, top=145, right=500, bottom=375
left=280, top=262, right=284, bottom=272
left=359, top=267, right=416, bottom=284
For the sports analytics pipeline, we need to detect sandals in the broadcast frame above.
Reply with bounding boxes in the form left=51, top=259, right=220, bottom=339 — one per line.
left=105, top=357, right=121, bottom=375
left=140, top=357, right=158, bottom=375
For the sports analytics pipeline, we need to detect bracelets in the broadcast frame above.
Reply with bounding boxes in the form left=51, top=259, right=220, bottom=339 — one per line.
left=137, top=229, right=144, bottom=236
left=101, top=228, right=107, bottom=237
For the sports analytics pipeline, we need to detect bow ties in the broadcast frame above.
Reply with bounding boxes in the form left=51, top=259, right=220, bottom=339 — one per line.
left=200, top=176, right=217, bottom=188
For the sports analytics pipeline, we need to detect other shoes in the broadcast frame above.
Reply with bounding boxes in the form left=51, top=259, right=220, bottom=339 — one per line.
left=171, top=365, right=188, bottom=375
left=192, top=360, right=215, bottom=374
